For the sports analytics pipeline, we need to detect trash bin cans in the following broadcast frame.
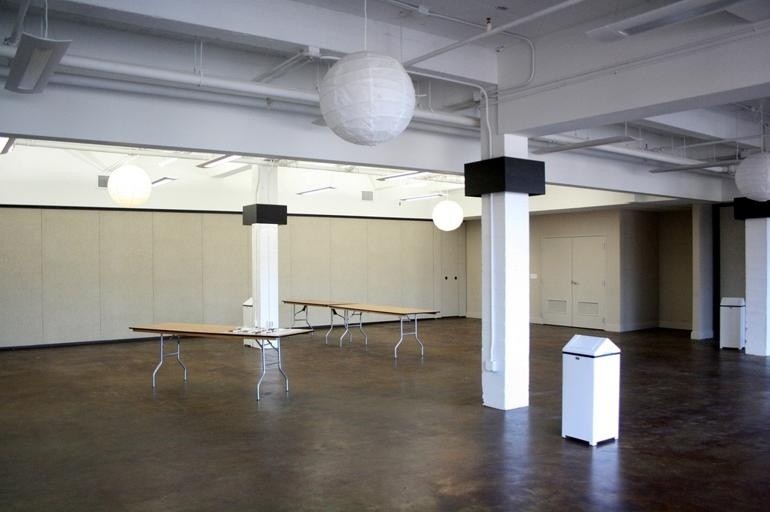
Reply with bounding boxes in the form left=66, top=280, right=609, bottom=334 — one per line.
left=563, top=334, right=620, bottom=445
left=719, top=297, right=746, bottom=352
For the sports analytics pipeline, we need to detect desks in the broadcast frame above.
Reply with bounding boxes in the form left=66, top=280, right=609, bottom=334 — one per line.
left=129, top=322, right=313, bottom=398
left=282, top=295, right=439, bottom=364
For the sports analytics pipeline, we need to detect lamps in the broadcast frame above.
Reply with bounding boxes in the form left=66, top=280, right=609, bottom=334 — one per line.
left=431, top=173, right=465, bottom=232
left=316, top=2, right=417, bottom=149
left=107, top=153, right=152, bottom=209
left=733, top=102, right=770, bottom=202
